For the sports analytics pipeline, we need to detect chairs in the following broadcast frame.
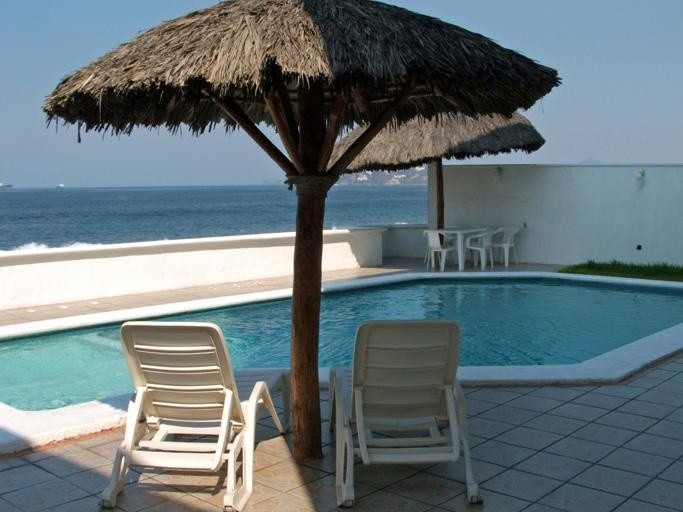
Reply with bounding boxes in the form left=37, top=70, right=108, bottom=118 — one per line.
left=98, top=319, right=290, bottom=510
left=423, top=226, right=520, bottom=272
left=327, top=320, right=479, bottom=509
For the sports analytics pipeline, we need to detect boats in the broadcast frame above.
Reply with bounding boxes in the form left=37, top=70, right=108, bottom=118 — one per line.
left=0, top=182, right=12, bottom=188
left=56, top=183, right=64, bottom=189
